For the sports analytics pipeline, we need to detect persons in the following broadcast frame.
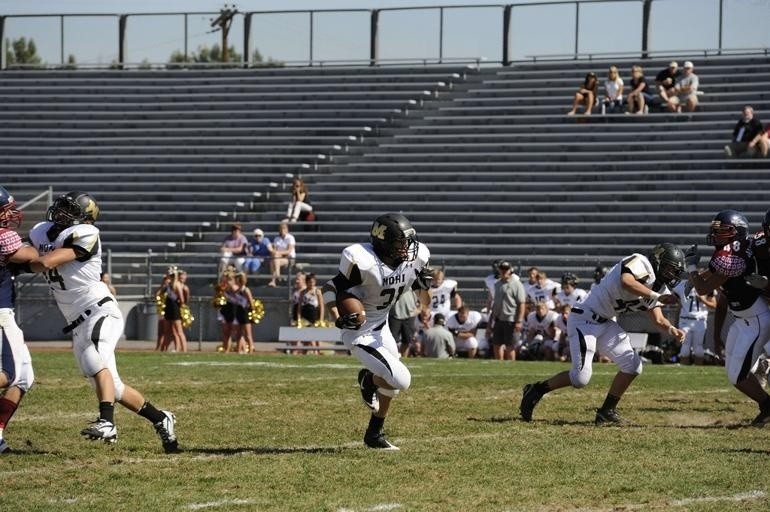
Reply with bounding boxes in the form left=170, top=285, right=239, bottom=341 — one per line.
left=673, top=267, right=717, bottom=364
left=520, top=242, right=687, bottom=428
left=281, top=178, right=312, bottom=222
left=744, top=210, right=770, bottom=304
left=388, top=260, right=610, bottom=363
left=567, top=61, right=699, bottom=115
left=99, top=272, right=116, bottom=296
left=686, top=210, right=770, bottom=427
left=724, top=106, right=770, bottom=158
left=322, top=213, right=437, bottom=452
left=213, top=223, right=349, bottom=354
left=0, top=185, right=41, bottom=455
left=8, top=191, right=178, bottom=454
left=156, top=266, right=194, bottom=352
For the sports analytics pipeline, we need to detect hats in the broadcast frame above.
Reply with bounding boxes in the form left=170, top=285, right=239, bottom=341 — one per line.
left=684, top=61, right=692, bottom=68
left=253, top=228, right=263, bottom=235
left=688, top=264, right=698, bottom=273
left=669, top=61, right=678, bottom=67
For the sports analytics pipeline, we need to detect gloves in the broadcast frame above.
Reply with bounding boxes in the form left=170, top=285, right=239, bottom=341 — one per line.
left=415, top=266, right=434, bottom=291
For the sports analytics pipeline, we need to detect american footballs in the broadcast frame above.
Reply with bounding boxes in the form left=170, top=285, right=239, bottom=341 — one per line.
left=336, top=291, right=367, bottom=326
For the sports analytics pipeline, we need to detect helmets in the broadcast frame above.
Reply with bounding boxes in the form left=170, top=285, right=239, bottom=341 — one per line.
left=0, top=185, right=25, bottom=230
left=492, top=258, right=515, bottom=284
left=706, top=210, right=749, bottom=246
left=370, top=213, right=418, bottom=262
left=650, top=242, right=686, bottom=288
left=46, top=191, right=100, bottom=227
left=560, top=264, right=608, bottom=284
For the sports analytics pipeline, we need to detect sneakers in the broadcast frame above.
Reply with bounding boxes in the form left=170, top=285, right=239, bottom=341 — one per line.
left=593, top=406, right=629, bottom=425
left=519, top=381, right=541, bottom=421
left=81, top=417, right=118, bottom=445
left=363, top=429, right=400, bottom=451
left=152, top=409, right=178, bottom=452
left=358, top=369, right=380, bottom=413
left=751, top=411, right=770, bottom=425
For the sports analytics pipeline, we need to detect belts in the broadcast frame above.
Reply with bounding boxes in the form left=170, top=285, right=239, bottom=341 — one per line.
left=373, top=320, right=386, bottom=331
left=681, top=316, right=697, bottom=320
left=571, top=307, right=608, bottom=323
left=62, top=297, right=112, bottom=333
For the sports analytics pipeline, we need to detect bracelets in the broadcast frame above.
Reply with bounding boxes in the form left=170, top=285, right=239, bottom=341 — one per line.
left=687, top=265, right=698, bottom=273
left=650, top=290, right=661, bottom=301
left=669, top=326, right=675, bottom=335
